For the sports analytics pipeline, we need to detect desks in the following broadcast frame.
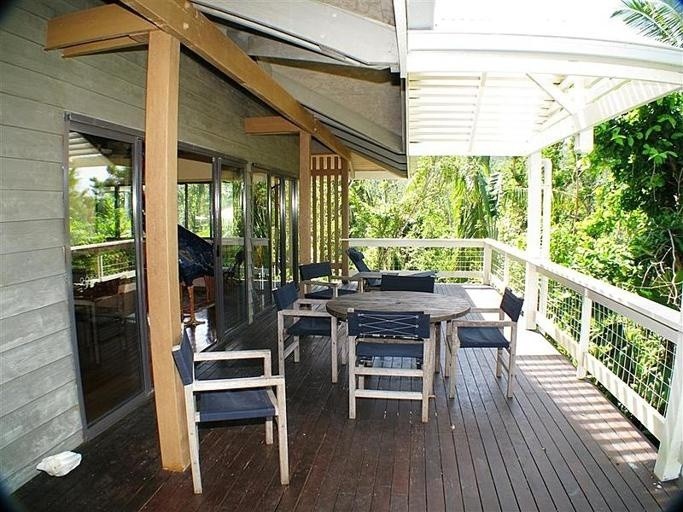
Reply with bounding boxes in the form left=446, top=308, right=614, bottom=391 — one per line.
left=350, top=271, right=431, bottom=295
left=326, top=290, right=471, bottom=374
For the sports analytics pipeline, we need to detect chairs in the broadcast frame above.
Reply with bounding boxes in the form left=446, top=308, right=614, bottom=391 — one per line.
left=348, top=308, right=435, bottom=424
left=271, top=282, right=347, bottom=382
left=366, top=275, right=434, bottom=369
left=172, top=324, right=289, bottom=494
left=345, top=247, right=398, bottom=292
left=73, top=278, right=126, bottom=365
left=444, top=289, right=524, bottom=400
left=298, top=262, right=363, bottom=310
